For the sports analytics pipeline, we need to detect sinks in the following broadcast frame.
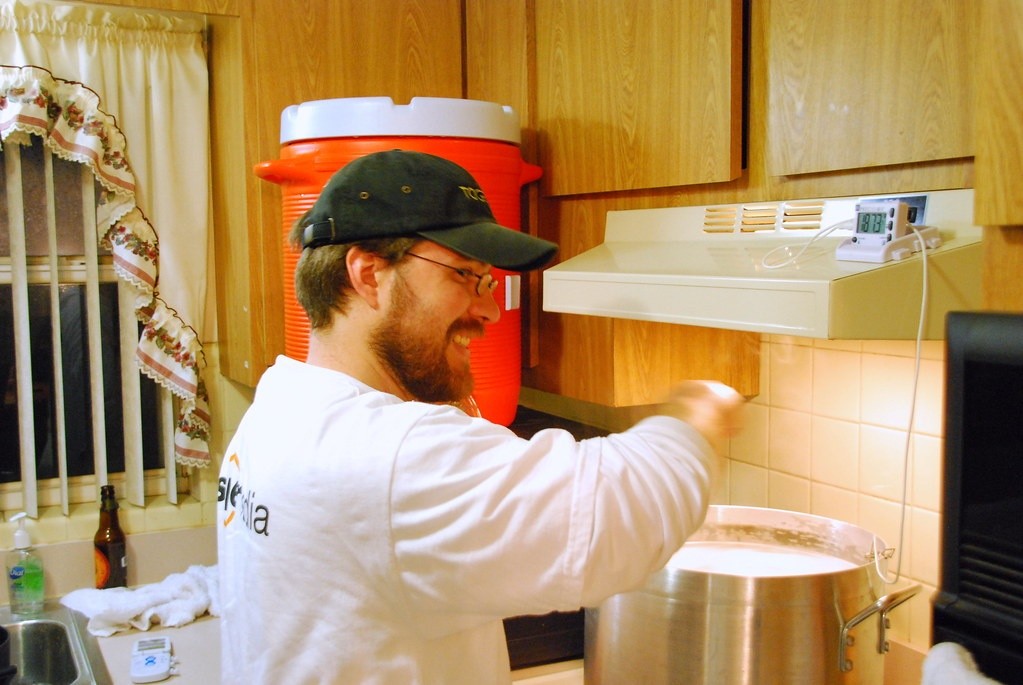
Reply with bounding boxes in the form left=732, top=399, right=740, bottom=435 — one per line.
left=0, top=608, right=96, bottom=685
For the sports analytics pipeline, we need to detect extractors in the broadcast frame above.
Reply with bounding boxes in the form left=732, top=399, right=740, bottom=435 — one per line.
left=543, top=188, right=983, bottom=339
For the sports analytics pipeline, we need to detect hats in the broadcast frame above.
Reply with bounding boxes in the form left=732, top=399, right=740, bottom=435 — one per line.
left=300, top=149, right=559, bottom=272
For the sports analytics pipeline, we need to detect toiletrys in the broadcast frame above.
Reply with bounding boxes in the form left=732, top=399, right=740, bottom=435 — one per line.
left=6, top=512, right=43, bottom=615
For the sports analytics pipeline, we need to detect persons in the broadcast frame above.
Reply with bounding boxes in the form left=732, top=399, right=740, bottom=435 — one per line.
left=217, top=149, right=749, bottom=685
left=7, top=285, right=114, bottom=479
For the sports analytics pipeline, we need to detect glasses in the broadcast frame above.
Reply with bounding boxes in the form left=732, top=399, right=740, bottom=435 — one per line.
left=402, top=251, right=498, bottom=297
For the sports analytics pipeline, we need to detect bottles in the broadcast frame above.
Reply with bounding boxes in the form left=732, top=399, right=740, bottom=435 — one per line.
left=93, top=485, right=127, bottom=590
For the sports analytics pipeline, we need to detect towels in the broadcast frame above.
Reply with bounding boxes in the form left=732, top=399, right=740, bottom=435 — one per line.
left=60, top=563, right=221, bottom=638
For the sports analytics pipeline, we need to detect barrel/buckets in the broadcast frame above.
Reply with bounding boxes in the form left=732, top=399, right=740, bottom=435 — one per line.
left=255, top=96, right=543, bottom=428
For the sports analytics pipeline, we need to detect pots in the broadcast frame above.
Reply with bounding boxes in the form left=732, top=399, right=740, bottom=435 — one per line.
left=585, top=505, right=922, bottom=685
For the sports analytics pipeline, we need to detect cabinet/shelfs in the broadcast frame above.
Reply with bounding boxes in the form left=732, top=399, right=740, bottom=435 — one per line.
left=463, top=0, right=1023, bottom=408
left=208, top=0, right=466, bottom=386
